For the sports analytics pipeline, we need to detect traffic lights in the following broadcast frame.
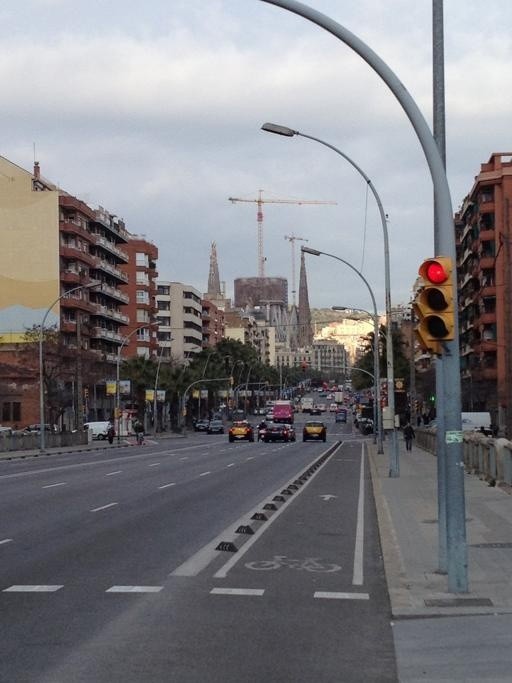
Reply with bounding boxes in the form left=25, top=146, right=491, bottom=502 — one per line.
left=419, top=256, right=455, bottom=343
left=302, top=363, right=306, bottom=372
left=85, top=389, right=89, bottom=398
left=114, top=407, right=123, bottom=418
left=411, top=296, right=439, bottom=353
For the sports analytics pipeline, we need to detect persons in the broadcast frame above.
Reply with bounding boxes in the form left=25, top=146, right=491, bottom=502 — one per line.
left=132, top=415, right=144, bottom=442
left=490, top=421, right=498, bottom=436
left=108, top=428, right=114, bottom=444
left=407, top=394, right=436, bottom=427
left=404, top=423, right=416, bottom=451
left=191, top=416, right=197, bottom=432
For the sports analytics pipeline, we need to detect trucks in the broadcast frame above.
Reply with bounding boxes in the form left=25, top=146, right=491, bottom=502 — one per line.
left=71, top=421, right=111, bottom=439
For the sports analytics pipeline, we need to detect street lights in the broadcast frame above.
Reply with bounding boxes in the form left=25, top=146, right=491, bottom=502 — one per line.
left=177, top=345, right=200, bottom=429
left=301, top=244, right=385, bottom=455
left=152, top=336, right=175, bottom=432
left=332, top=304, right=378, bottom=444
left=115, top=320, right=163, bottom=442
left=38, top=280, right=104, bottom=452
left=261, top=119, right=400, bottom=479
left=201, top=352, right=258, bottom=419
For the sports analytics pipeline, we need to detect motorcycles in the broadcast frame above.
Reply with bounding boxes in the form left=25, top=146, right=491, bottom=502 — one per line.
left=136, top=431, right=145, bottom=445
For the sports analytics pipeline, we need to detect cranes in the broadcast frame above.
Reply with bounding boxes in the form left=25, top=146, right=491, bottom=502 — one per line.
left=227, top=188, right=340, bottom=276
left=284, top=231, right=310, bottom=302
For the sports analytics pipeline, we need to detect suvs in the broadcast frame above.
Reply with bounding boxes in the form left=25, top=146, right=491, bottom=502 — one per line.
left=13, top=423, right=59, bottom=437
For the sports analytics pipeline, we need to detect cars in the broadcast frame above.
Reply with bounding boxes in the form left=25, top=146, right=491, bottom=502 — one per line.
left=425, top=411, right=493, bottom=437
left=194, top=380, right=373, bottom=443
left=0, top=425, right=13, bottom=435
